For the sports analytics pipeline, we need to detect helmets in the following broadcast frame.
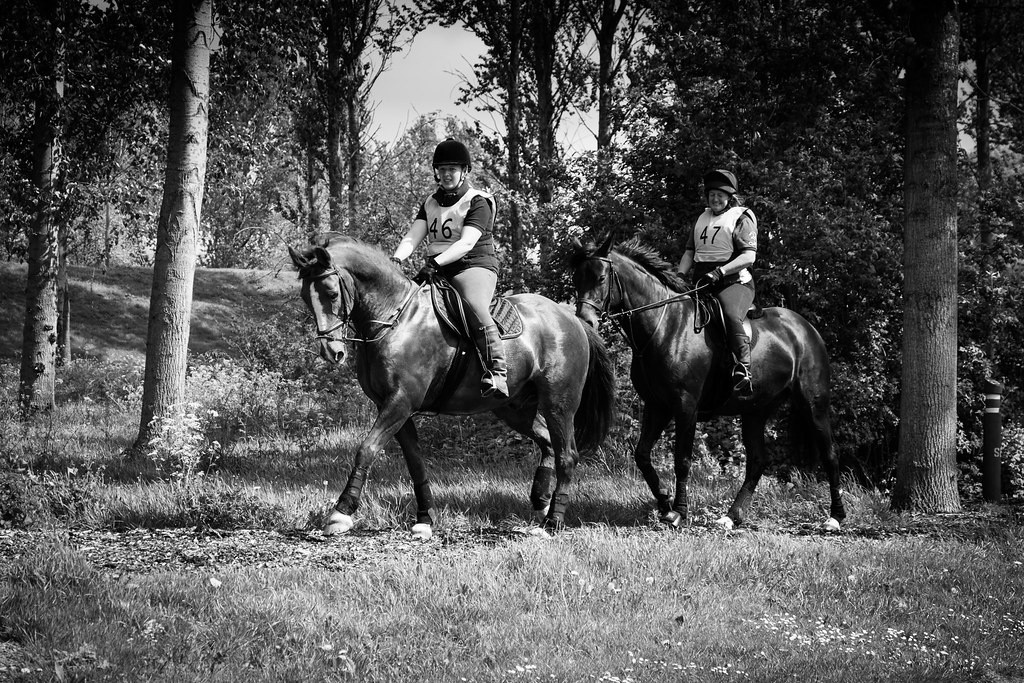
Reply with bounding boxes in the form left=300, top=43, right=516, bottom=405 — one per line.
left=432, top=137, right=471, bottom=172
left=704, top=169, right=738, bottom=197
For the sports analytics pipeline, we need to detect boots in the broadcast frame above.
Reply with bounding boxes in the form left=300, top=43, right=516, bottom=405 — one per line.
left=472, top=324, right=510, bottom=399
left=728, top=334, right=753, bottom=401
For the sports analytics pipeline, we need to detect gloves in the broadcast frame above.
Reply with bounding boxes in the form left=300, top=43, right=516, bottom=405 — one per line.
left=416, top=258, right=440, bottom=285
left=699, top=266, right=724, bottom=286
left=677, top=273, right=685, bottom=281
left=390, top=256, right=401, bottom=266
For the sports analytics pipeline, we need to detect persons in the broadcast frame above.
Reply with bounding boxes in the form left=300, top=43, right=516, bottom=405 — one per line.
left=676, top=169, right=758, bottom=396
left=391, top=137, right=509, bottom=399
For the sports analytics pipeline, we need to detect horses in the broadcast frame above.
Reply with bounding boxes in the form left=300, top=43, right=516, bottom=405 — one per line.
left=566, top=233, right=845, bottom=536
left=288, top=233, right=616, bottom=542
